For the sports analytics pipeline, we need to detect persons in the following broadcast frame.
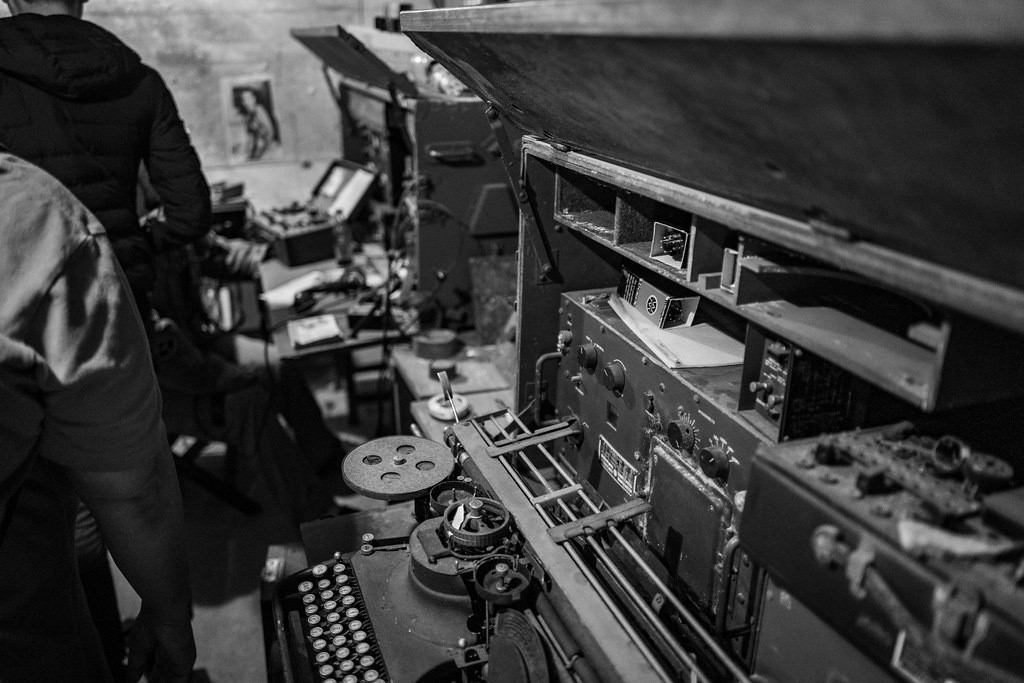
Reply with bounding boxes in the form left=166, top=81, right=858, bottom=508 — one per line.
left=0, top=0, right=213, bottom=609
left=223, top=79, right=280, bottom=161
left=0, top=151, right=244, bottom=683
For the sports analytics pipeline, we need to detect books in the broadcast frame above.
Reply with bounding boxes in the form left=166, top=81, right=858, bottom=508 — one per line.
left=604, top=294, right=748, bottom=369
left=287, top=312, right=345, bottom=351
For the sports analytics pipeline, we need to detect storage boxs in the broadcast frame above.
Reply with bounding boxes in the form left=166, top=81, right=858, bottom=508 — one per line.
left=251, top=156, right=383, bottom=269
